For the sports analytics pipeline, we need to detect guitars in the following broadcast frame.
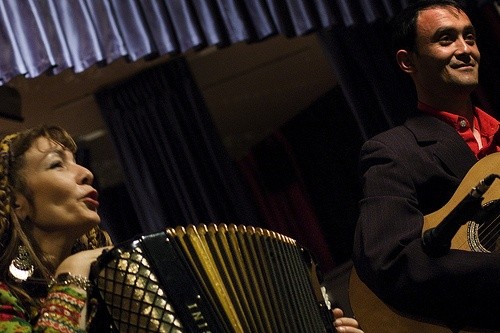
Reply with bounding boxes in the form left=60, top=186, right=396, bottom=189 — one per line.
left=349, top=150, right=500, bottom=332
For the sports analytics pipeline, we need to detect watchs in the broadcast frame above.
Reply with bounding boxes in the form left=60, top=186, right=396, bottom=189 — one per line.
left=47, top=273, right=93, bottom=293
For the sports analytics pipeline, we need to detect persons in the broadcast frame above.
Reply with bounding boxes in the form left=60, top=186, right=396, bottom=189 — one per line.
left=352, top=1, right=500, bottom=327
left=0, top=123, right=365, bottom=333
